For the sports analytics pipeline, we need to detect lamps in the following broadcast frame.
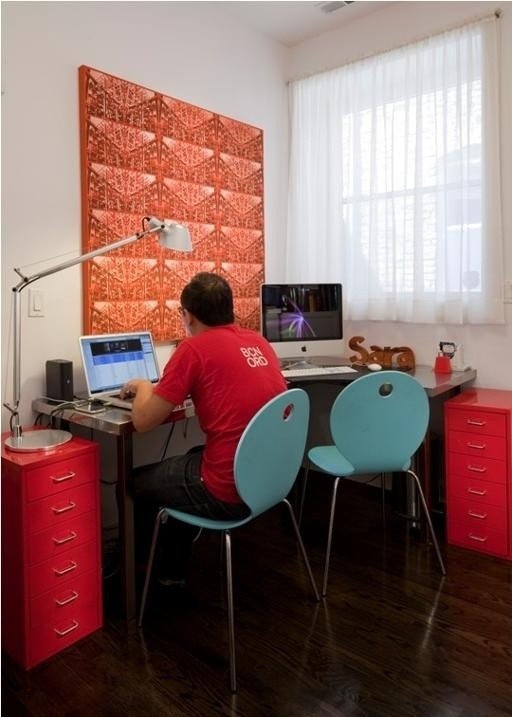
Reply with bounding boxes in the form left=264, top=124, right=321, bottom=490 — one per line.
left=6, top=214, right=195, bottom=455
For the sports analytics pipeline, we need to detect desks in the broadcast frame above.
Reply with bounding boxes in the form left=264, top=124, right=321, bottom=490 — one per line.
left=31, top=362, right=479, bottom=640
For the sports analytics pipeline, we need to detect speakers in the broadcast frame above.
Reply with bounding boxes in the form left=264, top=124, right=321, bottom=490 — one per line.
left=46, top=358, right=75, bottom=405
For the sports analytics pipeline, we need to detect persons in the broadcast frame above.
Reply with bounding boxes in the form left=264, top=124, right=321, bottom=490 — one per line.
left=118, top=272, right=288, bottom=585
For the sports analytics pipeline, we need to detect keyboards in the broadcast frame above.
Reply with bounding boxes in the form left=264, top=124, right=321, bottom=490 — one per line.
left=280, top=366, right=357, bottom=378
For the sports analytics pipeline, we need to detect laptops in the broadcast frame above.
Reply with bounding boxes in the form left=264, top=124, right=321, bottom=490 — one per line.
left=78, top=331, right=164, bottom=409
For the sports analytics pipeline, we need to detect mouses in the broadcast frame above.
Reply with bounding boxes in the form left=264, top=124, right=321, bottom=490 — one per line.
left=367, top=362, right=382, bottom=372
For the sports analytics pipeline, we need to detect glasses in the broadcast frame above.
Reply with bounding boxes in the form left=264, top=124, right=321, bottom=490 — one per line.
left=178, top=307, right=185, bottom=316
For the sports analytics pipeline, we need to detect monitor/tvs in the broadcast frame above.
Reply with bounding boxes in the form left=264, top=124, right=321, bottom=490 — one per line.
left=260, top=280, right=345, bottom=370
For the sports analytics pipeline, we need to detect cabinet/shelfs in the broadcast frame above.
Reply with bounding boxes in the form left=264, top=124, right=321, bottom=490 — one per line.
left=5, top=423, right=106, bottom=671
left=442, top=385, right=512, bottom=562
